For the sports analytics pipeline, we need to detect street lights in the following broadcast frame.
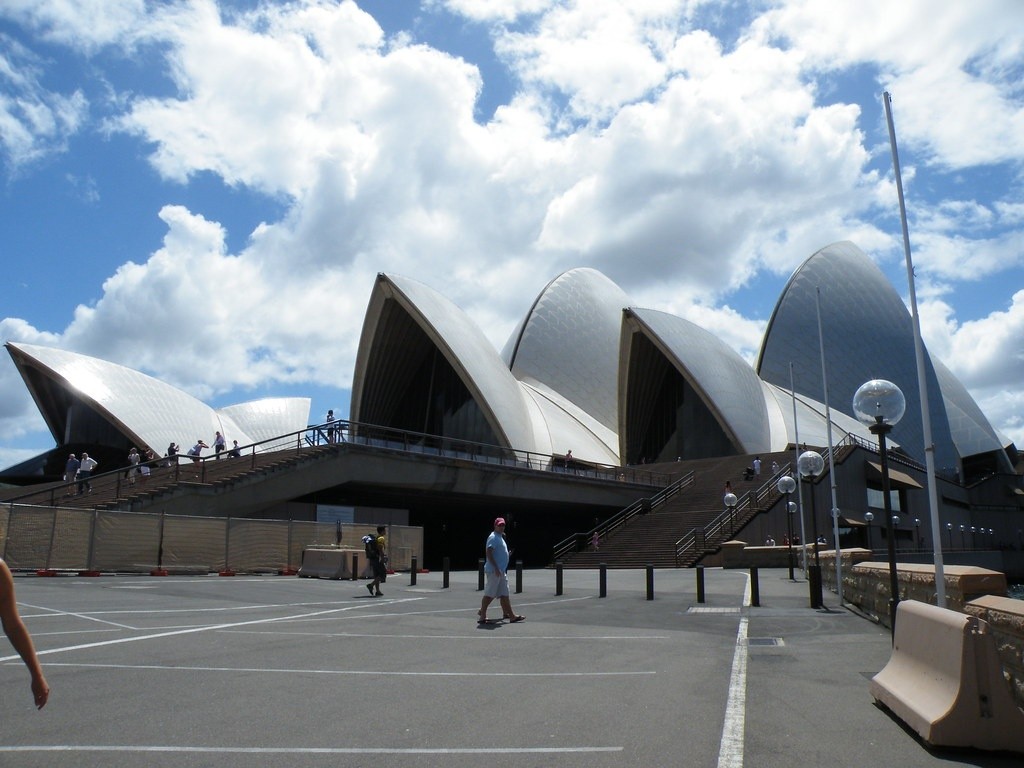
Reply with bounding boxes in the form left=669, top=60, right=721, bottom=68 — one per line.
left=969, top=527, right=977, bottom=546
left=980, top=528, right=985, bottom=547
left=796, top=450, right=825, bottom=609
left=865, top=511, right=875, bottom=549
left=945, top=522, right=954, bottom=550
left=988, top=529, right=994, bottom=547
left=777, top=476, right=796, bottom=580
left=784, top=502, right=797, bottom=545
left=912, top=518, right=922, bottom=553
left=958, top=524, right=966, bottom=551
left=723, top=492, right=738, bottom=540
left=853, top=378, right=908, bottom=648
left=892, top=515, right=901, bottom=550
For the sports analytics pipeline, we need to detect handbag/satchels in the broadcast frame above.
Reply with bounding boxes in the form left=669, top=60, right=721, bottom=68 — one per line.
left=187, top=449, right=195, bottom=459
left=220, top=444, right=224, bottom=450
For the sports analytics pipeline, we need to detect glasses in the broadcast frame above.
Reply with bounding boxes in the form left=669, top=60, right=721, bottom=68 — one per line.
left=497, top=524, right=505, bottom=527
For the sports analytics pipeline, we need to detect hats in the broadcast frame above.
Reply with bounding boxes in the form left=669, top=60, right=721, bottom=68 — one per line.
left=494, top=517, right=506, bottom=526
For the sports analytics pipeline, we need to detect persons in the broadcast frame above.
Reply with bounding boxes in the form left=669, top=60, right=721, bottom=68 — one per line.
left=592, top=532, right=599, bottom=552
left=367, top=525, right=388, bottom=597
left=145, top=448, right=154, bottom=469
left=0, top=557, right=49, bottom=710
left=752, top=456, right=762, bottom=482
left=723, top=480, right=732, bottom=496
left=65, top=453, right=80, bottom=496
left=190, top=440, right=209, bottom=478
left=226, top=440, right=241, bottom=460
left=124, top=447, right=140, bottom=488
left=771, top=462, right=779, bottom=475
left=478, top=518, right=526, bottom=624
left=168, top=443, right=184, bottom=479
left=211, top=431, right=225, bottom=460
left=565, top=450, right=572, bottom=474
left=162, top=453, right=169, bottom=471
left=78, top=452, right=97, bottom=495
left=817, top=533, right=826, bottom=543
left=327, top=410, right=335, bottom=445
left=765, top=534, right=775, bottom=546
left=783, top=533, right=800, bottom=546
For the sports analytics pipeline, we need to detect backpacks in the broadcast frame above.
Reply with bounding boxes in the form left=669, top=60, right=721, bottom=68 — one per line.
left=364, top=534, right=379, bottom=558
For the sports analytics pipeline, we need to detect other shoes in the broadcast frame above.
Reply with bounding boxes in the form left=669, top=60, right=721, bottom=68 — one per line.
left=89, top=487, right=92, bottom=494
left=366, top=584, right=373, bottom=595
left=478, top=610, right=482, bottom=615
left=502, top=614, right=510, bottom=619
left=375, top=591, right=384, bottom=595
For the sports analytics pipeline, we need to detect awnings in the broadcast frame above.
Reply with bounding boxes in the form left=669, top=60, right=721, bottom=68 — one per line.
left=836, top=506, right=870, bottom=526
left=866, top=458, right=923, bottom=489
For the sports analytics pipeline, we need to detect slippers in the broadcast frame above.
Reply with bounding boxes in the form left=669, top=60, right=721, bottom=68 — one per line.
left=478, top=618, right=493, bottom=625
left=510, top=615, right=526, bottom=623
